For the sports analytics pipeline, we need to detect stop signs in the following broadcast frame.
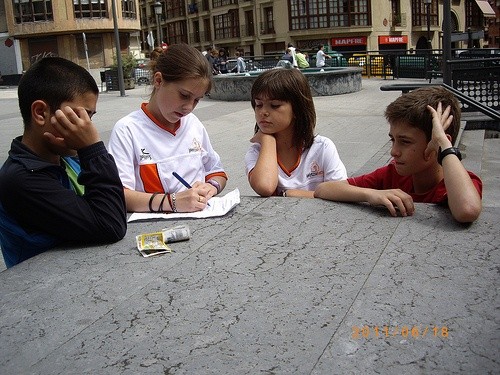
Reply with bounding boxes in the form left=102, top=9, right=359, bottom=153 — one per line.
left=162, top=43, right=168, bottom=50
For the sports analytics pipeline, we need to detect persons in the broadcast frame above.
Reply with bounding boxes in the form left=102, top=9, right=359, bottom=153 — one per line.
left=206, top=47, right=229, bottom=74
left=313, top=88, right=483, bottom=222
left=245, top=68, right=348, bottom=199
left=280, top=43, right=310, bottom=68
left=0, top=56, right=127, bottom=269
left=108, top=43, right=228, bottom=213
left=316, top=45, right=331, bottom=67
left=230, top=52, right=247, bottom=72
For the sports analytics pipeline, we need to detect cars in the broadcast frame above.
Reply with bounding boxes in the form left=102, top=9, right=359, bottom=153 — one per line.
left=133, top=56, right=443, bottom=86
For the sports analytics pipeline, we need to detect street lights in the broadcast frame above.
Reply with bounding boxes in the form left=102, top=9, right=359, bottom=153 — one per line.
left=154, top=2, right=162, bottom=46
left=423, top=0, right=432, bottom=56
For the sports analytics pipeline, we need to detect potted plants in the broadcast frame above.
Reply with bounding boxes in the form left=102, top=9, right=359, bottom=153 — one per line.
left=110, top=49, right=138, bottom=90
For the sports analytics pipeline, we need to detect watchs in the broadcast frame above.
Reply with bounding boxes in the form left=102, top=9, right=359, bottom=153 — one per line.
left=206, top=179, right=220, bottom=193
left=437, top=146, right=462, bottom=166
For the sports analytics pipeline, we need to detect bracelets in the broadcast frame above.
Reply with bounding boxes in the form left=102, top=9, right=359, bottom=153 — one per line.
left=149, top=192, right=160, bottom=213
left=168, top=192, right=173, bottom=210
left=158, top=192, right=168, bottom=213
left=172, top=192, right=177, bottom=212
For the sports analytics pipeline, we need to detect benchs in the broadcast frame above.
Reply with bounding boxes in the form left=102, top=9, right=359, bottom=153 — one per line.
left=208, top=67, right=365, bottom=101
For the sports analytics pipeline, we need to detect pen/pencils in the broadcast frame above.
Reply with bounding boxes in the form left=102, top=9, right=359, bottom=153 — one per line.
left=172, top=172, right=210, bottom=206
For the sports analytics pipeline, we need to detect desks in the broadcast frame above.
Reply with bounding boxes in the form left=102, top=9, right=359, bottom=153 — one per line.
left=0, top=195, right=500, bottom=375
